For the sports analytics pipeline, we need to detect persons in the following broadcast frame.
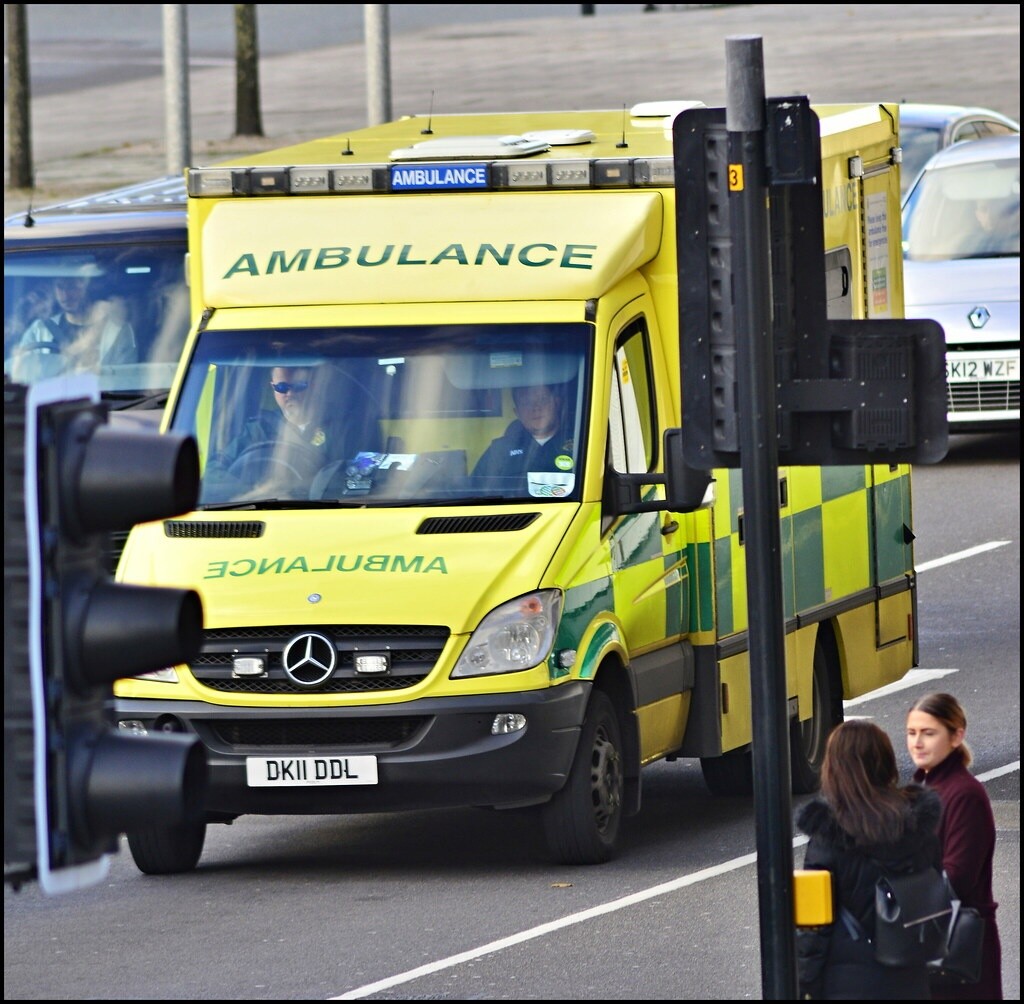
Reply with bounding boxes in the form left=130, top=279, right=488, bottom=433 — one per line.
left=939, top=189, right=1020, bottom=256
left=463, top=377, right=576, bottom=498
left=198, top=367, right=382, bottom=504
left=793, top=719, right=942, bottom=1001
left=908, top=691, right=1003, bottom=1003
left=11, top=278, right=137, bottom=385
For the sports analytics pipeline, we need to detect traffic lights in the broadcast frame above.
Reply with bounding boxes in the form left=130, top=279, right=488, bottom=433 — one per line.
left=0, top=378, right=207, bottom=896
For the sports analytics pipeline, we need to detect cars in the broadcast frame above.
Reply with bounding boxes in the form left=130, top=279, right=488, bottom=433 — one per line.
left=892, top=104, right=1021, bottom=201
left=901, top=138, right=1019, bottom=431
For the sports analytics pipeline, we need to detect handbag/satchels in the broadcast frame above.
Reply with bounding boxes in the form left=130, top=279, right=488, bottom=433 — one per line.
left=929, top=908, right=986, bottom=987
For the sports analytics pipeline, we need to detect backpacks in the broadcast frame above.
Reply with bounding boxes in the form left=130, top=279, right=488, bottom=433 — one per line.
left=838, top=869, right=953, bottom=969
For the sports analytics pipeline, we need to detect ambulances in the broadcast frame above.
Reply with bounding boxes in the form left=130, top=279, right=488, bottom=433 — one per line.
left=111, top=95, right=921, bottom=875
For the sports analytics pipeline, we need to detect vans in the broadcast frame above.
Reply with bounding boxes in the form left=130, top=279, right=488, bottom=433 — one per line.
left=4, top=169, right=190, bottom=387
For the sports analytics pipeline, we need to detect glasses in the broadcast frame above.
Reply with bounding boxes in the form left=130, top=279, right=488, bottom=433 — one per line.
left=270, top=380, right=309, bottom=393
